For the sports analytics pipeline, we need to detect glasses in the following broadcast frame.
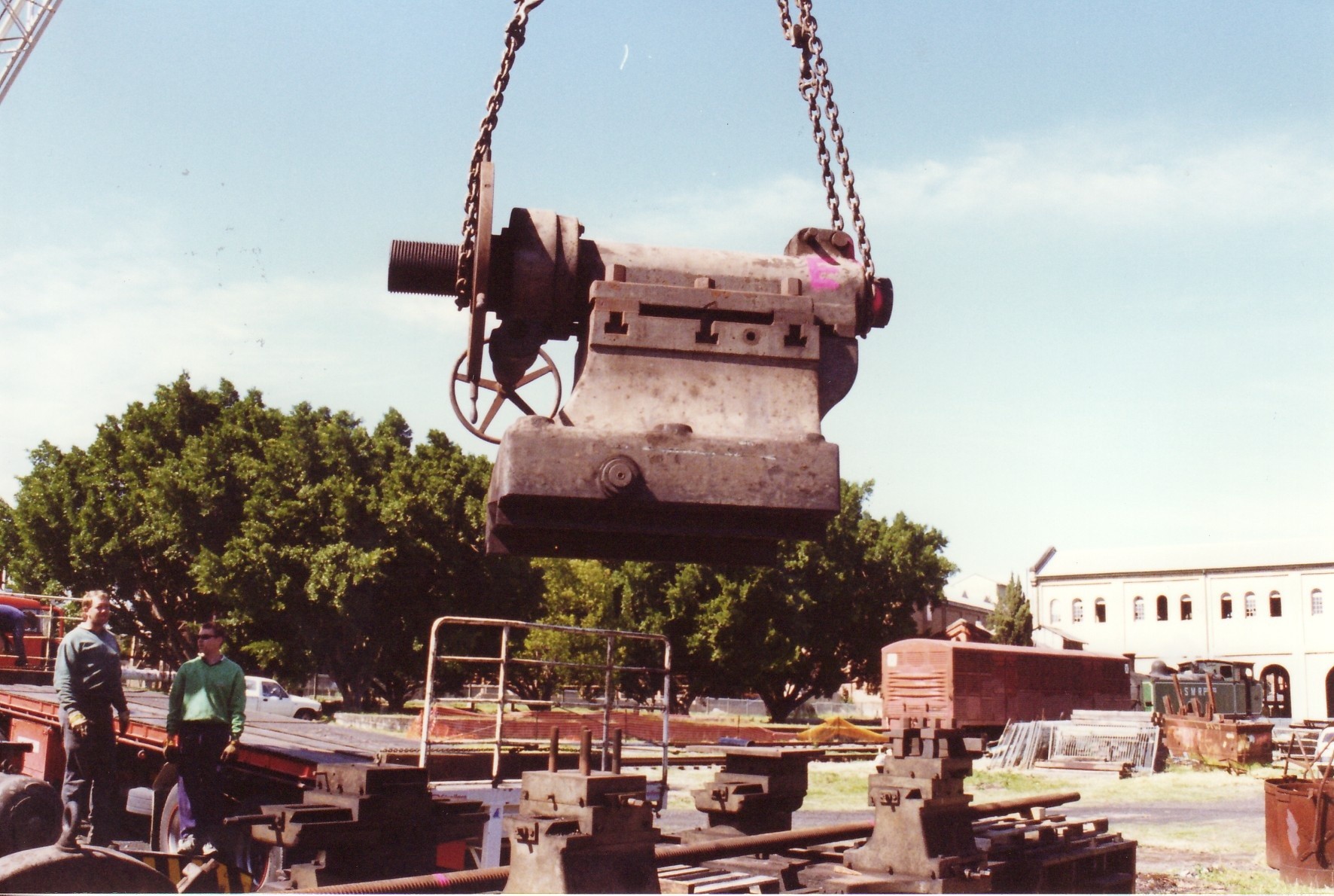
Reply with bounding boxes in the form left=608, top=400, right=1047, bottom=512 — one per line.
left=195, top=633, right=216, bottom=641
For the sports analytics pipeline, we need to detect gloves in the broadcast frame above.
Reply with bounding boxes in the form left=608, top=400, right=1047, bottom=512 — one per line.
left=118, top=711, right=130, bottom=737
left=68, top=711, right=94, bottom=737
left=162, top=742, right=179, bottom=763
left=220, top=740, right=239, bottom=764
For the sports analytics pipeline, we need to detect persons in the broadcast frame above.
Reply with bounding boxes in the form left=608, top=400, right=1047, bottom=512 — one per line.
left=163, top=621, right=247, bottom=856
left=0, top=604, right=39, bottom=664
left=52, top=590, right=131, bottom=851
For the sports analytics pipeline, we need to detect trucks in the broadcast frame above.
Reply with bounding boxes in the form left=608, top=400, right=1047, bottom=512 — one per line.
left=1, top=591, right=673, bottom=893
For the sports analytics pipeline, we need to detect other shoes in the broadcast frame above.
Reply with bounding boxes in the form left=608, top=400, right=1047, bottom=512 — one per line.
left=198, top=838, right=218, bottom=856
left=177, top=834, right=196, bottom=853
left=86, top=832, right=120, bottom=851
